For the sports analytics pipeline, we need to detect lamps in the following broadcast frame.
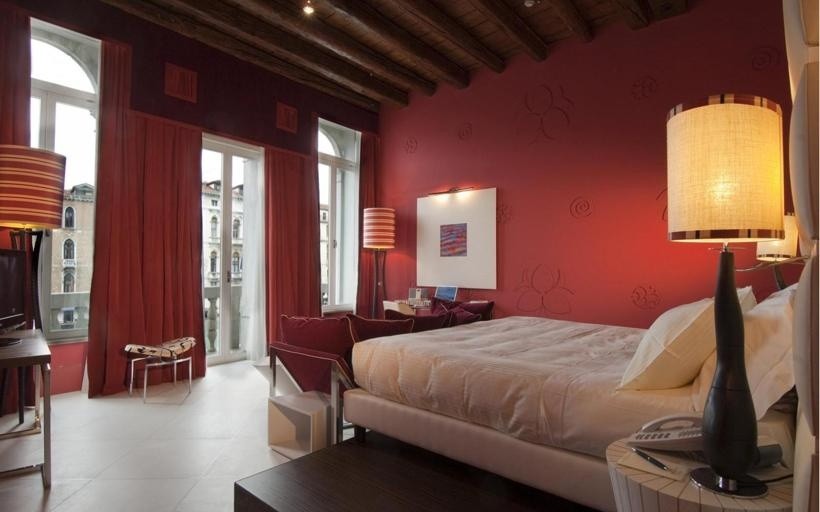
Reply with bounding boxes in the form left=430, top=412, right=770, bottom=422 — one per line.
left=363, top=207, right=395, bottom=315
left=664, top=90, right=790, bottom=499
left=302, top=0, right=314, bottom=13
left=756, top=211, right=799, bottom=290
left=0, top=145, right=66, bottom=330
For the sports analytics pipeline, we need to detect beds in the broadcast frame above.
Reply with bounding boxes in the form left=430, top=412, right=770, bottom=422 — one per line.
left=343, top=315, right=790, bottom=512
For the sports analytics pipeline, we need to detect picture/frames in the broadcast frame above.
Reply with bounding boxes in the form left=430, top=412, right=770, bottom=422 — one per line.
left=416, top=187, right=497, bottom=289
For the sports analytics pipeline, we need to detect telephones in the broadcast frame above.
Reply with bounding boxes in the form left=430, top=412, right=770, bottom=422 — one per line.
left=626, top=412, right=703, bottom=452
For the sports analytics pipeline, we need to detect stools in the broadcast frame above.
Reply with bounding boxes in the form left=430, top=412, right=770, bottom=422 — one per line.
left=125, top=336, right=196, bottom=404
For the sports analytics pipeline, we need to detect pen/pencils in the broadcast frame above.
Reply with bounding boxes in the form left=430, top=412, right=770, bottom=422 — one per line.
left=628, top=446, right=669, bottom=473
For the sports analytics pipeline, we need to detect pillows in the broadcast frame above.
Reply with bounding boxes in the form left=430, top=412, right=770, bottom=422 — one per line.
left=433, top=304, right=447, bottom=313
left=431, top=298, right=461, bottom=314
left=691, top=283, right=798, bottom=421
left=449, top=305, right=480, bottom=324
left=280, top=314, right=359, bottom=360
left=616, top=283, right=757, bottom=390
left=346, top=312, right=414, bottom=343
left=459, top=301, right=495, bottom=319
left=384, top=309, right=453, bottom=332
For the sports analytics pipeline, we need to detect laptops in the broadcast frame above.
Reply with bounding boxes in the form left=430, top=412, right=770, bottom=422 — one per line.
left=423, top=286, right=458, bottom=305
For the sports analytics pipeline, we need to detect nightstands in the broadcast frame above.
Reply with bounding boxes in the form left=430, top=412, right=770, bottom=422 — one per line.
left=605, top=435, right=792, bottom=512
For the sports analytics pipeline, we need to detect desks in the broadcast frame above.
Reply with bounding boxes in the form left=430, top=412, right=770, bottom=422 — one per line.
left=0, top=328, right=51, bottom=489
